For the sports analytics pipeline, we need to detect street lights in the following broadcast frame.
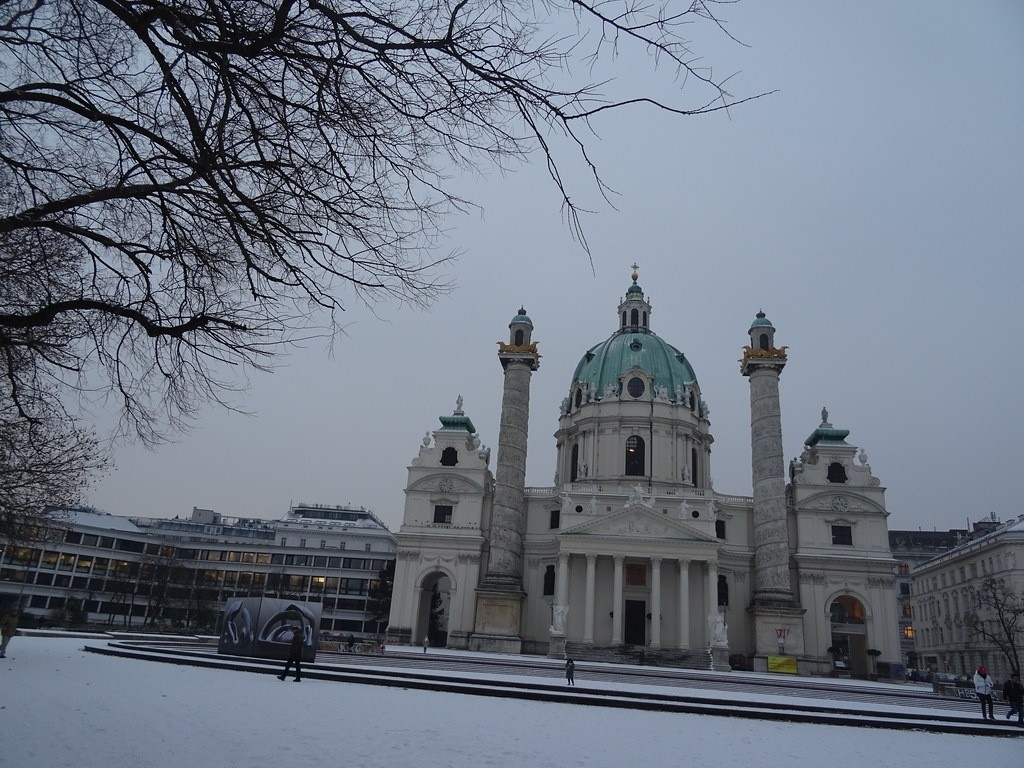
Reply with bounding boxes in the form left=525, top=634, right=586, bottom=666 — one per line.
left=213, top=613, right=222, bottom=636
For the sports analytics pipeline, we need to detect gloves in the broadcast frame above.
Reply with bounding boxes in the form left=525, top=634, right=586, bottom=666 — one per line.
left=1003, top=697, right=1007, bottom=700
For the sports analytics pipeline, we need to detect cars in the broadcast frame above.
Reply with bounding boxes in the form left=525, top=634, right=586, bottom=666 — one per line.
left=319, top=631, right=329, bottom=635
left=906, top=669, right=976, bottom=689
left=333, top=632, right=343, bottom=636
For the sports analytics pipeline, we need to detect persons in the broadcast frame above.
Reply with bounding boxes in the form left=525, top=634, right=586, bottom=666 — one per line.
left=631, top=483, right=643, bottom=501
left=974, top=666, right=995, bottom=719
left=639, top=649, right=645, bottom=665
left=679, top=500, right=689, bottom=518
left=822, top=407, right=828, bottom=422
left=276, top=626, right=303, bottom=682
left=38, top=615, right=45, bottom=629
left=967, top=673, right=971, bottom=681
left=0, top=607, right=18, bottom=658
left=1003, top=674, right=1024, bottom=728
left=565, top=658, right=575, bottom=685
left=590, top=496, right=597, bottom=513
left=422, top=635, right=429, bottom=653
left=954, top=675, right=962, bottom=680
left=348, top=634, right=354, bottom=652
left=559, top=493, right=573, bottom=511
left=707, top=501, right=714, bottom=518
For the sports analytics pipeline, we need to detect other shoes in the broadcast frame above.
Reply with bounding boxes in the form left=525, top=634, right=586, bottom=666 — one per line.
left=293, top=679, right=300, bottom=682
left=989, top=715, right=996, bottom=721
left=1006, top=711, right=1010, bottom=719
left=983, top=717, right=987, bottom=720
left=0, top=655, right=6, bottom=658
left=277, top=676, right=284, bottom=680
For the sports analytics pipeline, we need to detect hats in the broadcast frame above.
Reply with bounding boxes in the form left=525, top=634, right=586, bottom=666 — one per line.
left=979, top=665, right=985, bottom=673
left=289, top=627, right=299, bottom=630
